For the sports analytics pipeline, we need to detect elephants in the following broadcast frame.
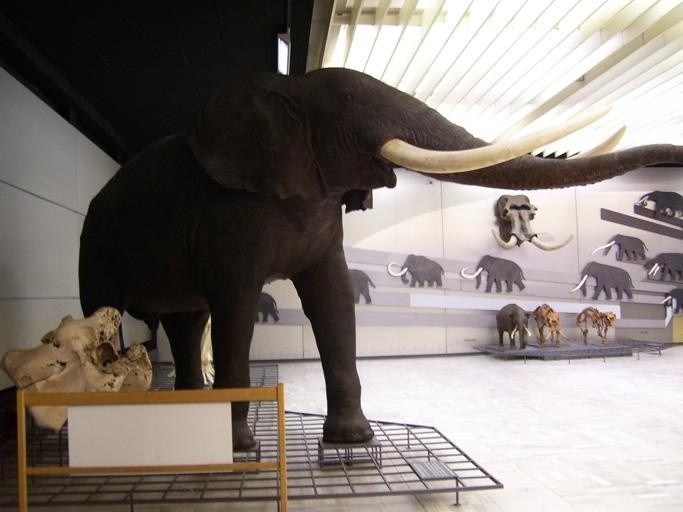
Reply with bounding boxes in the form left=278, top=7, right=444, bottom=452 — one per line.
left=495, top=302, right=533, bottom=351
left=69, top=61, right=681, bottom=454
